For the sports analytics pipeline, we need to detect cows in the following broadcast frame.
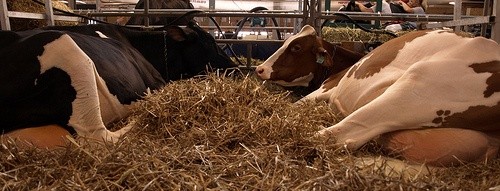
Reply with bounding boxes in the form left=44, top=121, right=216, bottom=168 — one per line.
left=0, top=19, right=239, bottom=150
left=124, top=0, right=196, bottom=26
left=255, top=25, right=500, bottom=178
left=330, top=0, right=408, bottom=29
